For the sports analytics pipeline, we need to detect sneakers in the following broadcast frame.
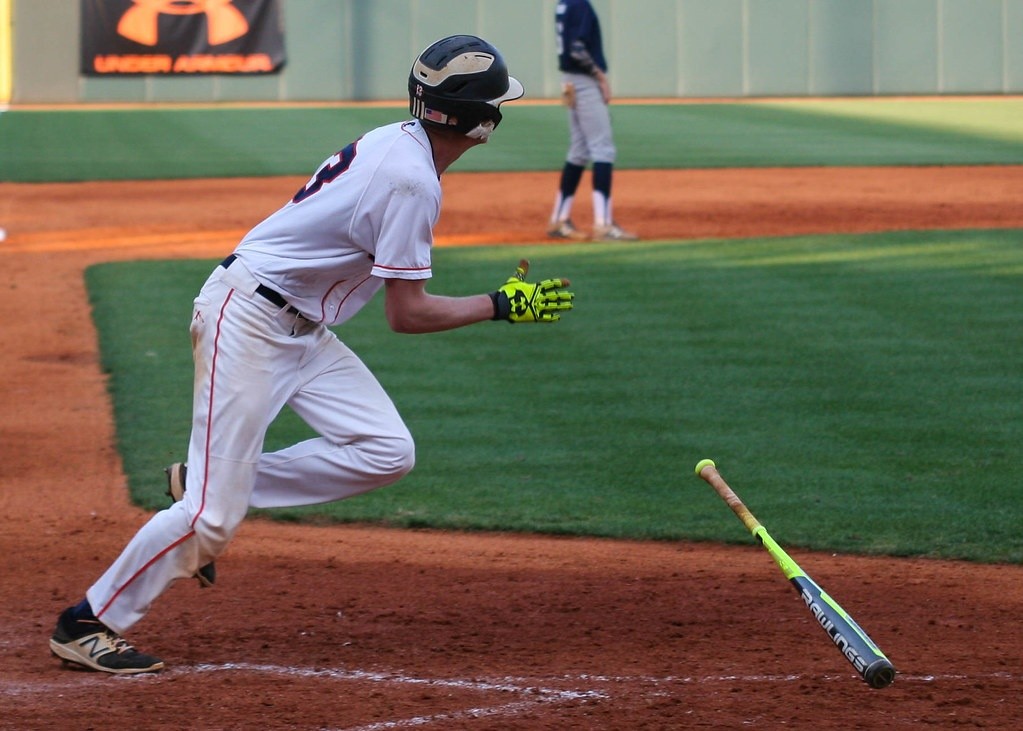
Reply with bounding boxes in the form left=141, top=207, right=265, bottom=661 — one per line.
left=163, top=462, right=215, bottom=588
left=592, top=224, right=637, bottom=241
left=546, top=219, right=588, bottom=241
left=49, top=606, right=164, bottom=674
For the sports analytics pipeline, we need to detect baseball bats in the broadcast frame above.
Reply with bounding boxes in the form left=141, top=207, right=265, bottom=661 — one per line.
left=693, top=458, right=896, bottom=689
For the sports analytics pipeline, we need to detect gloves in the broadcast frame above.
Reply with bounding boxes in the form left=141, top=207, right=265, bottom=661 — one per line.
left=488, top=259, right=575, bottom=323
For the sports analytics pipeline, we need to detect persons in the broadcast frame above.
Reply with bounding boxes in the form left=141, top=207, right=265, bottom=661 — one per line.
left=47, top=34, right=575, bottom=675
left=546, top=0, right=640, bottom=243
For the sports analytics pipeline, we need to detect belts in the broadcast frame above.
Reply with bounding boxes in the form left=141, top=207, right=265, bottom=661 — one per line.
left=219, top=253, right=306, bottom=319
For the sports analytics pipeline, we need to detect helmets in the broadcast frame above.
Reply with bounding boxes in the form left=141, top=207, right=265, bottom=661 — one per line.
left=408, top=35, right=524, bottom=143
